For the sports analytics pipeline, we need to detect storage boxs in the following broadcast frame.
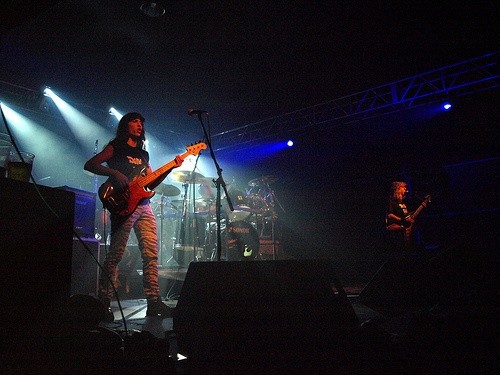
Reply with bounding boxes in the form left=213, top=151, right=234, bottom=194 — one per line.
left=70, top=237, right=100, bottom=297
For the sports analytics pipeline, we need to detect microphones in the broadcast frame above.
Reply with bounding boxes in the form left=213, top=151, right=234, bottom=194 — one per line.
left=188, top=107, right=208, bottom=115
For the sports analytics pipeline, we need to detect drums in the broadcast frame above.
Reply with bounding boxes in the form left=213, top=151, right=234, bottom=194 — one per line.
left=172, top=212, right=208, bottom=252
left=223, top=189, right=251, bottom=220
left=206, top=214, right=257, bottom=261
left=248, top=192, right=269, bottom=215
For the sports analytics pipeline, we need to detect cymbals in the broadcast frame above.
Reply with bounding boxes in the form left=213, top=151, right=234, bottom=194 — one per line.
left=152, top=182, right=181, bottom=197
left=171, top=171, right=205, bottom=184
left=247, top=174, right=280, bottom=187
left=202, top=176, right=217, bottom=188
left=169, top=199, right=197, bottom=211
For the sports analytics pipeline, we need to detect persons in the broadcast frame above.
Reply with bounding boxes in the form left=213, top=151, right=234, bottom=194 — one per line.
left=83, top=112, right=183, bottom=322
left=367, top=181, right=430, bottom=337
left=195, top=184, right=222, bottom=213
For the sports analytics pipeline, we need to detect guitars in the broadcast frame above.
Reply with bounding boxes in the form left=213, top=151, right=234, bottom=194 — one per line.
left=97, top=139, right=208, bottom=220
left=385, top=191, right=434, bottom=246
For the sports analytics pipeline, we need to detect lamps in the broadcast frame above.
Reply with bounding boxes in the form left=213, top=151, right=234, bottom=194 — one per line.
left=140, top=0, right=166, bottom=18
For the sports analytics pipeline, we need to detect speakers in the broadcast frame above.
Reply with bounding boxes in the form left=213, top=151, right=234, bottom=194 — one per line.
left=63, top=236, right=100, bottom=298
left=172, top=259, right=334, bottom=362
left=358, top=256, right=487, bottom=329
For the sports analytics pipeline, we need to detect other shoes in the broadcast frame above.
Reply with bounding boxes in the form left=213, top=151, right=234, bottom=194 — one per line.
left=99, top=297, right=114, bottom=322
left=146, top=297, right=175, bottom=317
